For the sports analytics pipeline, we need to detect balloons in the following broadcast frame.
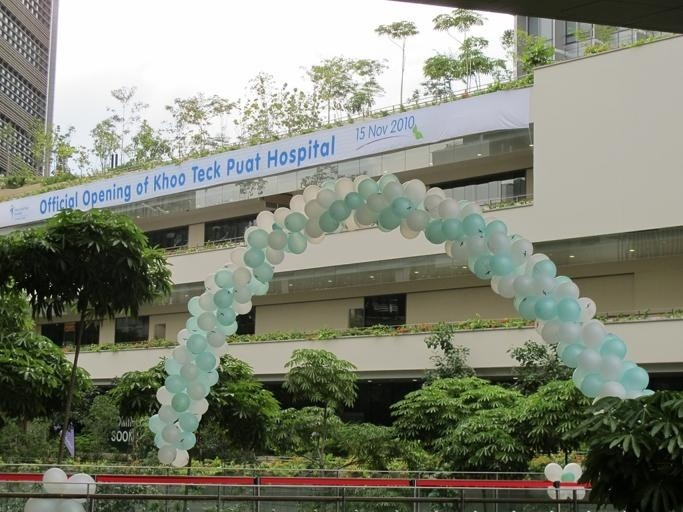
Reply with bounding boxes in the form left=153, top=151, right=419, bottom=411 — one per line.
left=544, top=461, right=585, bottom=499
left=187, top=275, right=252, bottom=348
left=149, top=405, right=201, bottom=469
left=43, top=467, right=67, bottom=495
left=303, top=174, right=427, bottom=240
left=24, top=498, right=56, bottom=512
left=156, top=329, right=228, bottom=414
left=57, top=497, right=86, bottom=512
left=66, top=473, right=96, bottom=503
left=513, top=253, right=654, bottom=404
left=423, top=187, right=534, bottom=299
left=215, top=195, right=308, bottom=288
